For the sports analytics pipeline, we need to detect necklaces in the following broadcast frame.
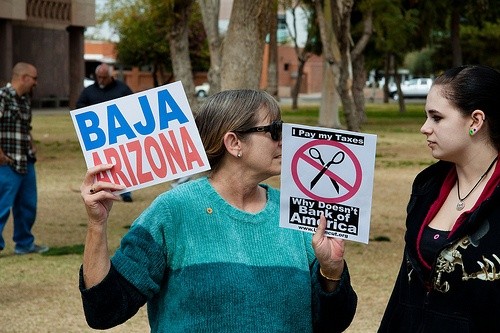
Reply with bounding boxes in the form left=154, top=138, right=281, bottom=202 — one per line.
left=456, top=156, right=498, bottom=211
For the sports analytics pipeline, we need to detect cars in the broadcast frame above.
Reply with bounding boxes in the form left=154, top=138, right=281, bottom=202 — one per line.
left=195, top=82, right=210, bottom=97
left=389, top=77, right=433, bottom=100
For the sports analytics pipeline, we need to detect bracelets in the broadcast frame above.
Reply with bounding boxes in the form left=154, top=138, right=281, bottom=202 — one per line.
left=320, top=268, right=341, bottom=281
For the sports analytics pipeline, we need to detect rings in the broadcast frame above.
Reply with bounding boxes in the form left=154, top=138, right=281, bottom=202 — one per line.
left=90, top=186, right=95, bottom=194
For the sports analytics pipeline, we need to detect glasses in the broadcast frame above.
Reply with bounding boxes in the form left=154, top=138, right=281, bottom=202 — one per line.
left=235, top=120, right=283, bottom=141
left=27, top=74, right=38, bottom=80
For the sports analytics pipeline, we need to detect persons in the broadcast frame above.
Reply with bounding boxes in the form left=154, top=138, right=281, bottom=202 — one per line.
left=369, top=70, right=377, bottom=101
left=79, top=88, right=357, bottom=333
left=0, top=62, right=49, bottom=254
left=75, top=64, right=133, bottom=202
left=378, top=65, right=500, bottom=333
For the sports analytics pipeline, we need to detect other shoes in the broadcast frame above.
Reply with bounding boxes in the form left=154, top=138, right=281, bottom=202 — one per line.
left=123, top=195, right=132, bottom=202
left=33, top=244, right=48, bottom=253
